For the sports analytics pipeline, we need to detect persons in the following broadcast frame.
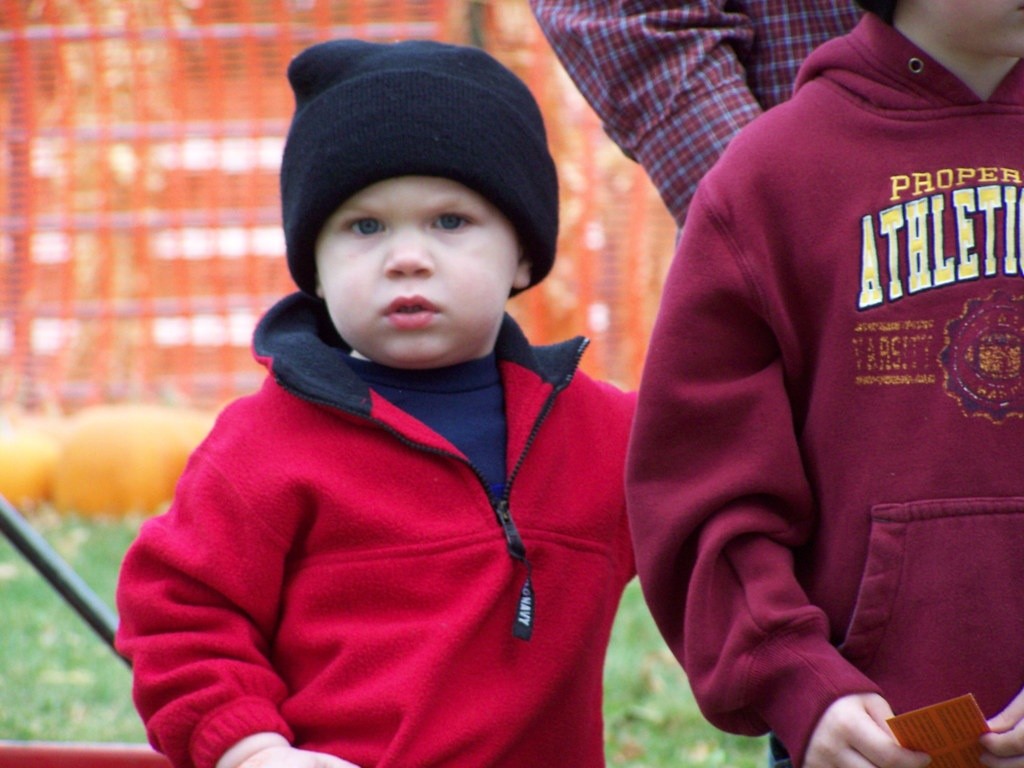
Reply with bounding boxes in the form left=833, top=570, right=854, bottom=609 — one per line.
left=628, top=0, right=1023, bottom=768
left=118, top=39, right=642, bottom=768
left=528, top=0, right=870, bottom=232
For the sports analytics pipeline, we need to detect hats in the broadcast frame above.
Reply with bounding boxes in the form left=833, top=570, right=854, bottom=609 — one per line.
left=280, top=39, right=559, bottom=300
